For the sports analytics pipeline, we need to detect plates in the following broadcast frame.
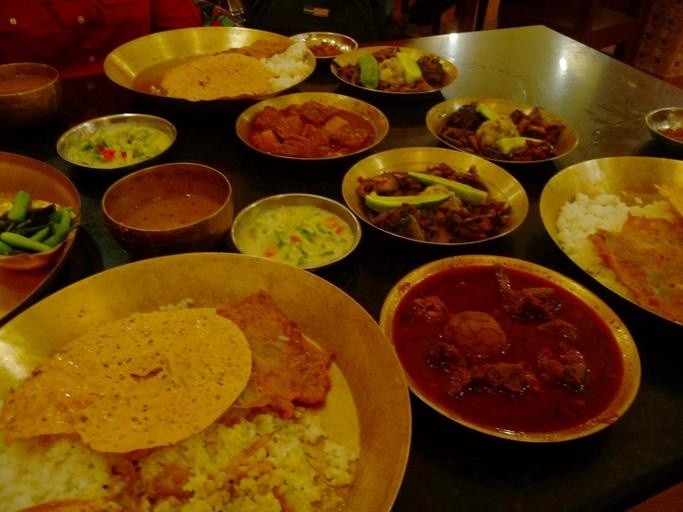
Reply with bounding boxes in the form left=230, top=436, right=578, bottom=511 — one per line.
left=236, top=90, right=391, bottom=163
left=331, top=44, right=459, bottom=97
left=378, top=255, right=643, bottom=444
left=340, top=147, right=532, bottom=249
left=102, top=28, right=319, bottom=103
left=424, top=94, right=581, bottom=169
left=1, top=150, right=82, bottom=323
left=290, top=32, right=358, bottom=60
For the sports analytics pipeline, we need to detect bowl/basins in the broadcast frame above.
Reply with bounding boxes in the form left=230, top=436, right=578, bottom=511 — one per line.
left=102, top=162, right=236, bottom=250
left=0, top=249, right=412, bottom=511
left=0, top=63, right=60, bottom=115
left=56, top=111, right=177, bottom=173
left=231, top=191, right=364, bottom=271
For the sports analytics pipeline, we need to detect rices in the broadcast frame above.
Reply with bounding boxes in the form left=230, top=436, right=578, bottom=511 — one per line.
left=555, top=185, right=672, bottom=303
left=258, top=41, right=312, bottom=94
left=0, top=410, right=353, bottom=512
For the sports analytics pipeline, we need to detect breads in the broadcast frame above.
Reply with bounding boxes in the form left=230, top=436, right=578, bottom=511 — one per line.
left=161, top=52, right=273, bottom=100
left=215, top=290, right=335, bottom=426
left=1, top=306, right=254, bottom=454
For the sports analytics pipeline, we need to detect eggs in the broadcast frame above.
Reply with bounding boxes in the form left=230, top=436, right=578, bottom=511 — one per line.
left=446, top=311, right=507, bottom=364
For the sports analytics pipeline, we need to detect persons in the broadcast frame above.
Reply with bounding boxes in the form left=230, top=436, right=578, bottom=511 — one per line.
left=1, top=0, right=204, bottom=83
